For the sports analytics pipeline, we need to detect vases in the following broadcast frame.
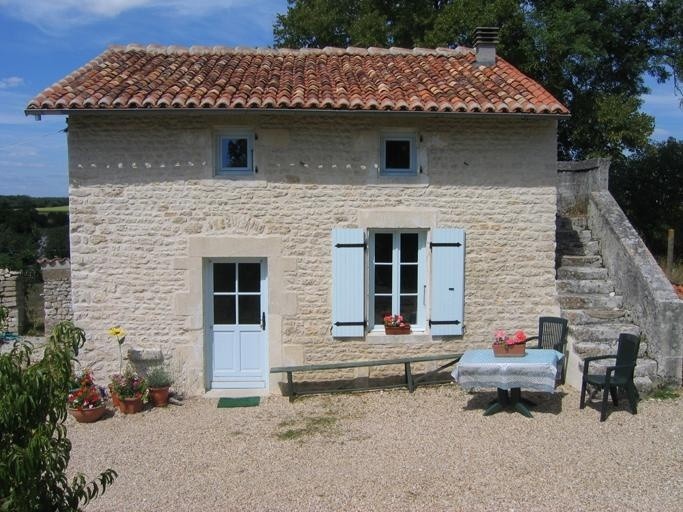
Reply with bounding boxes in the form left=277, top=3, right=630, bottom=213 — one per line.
left=68, top=406, right=106, bottom=423
left=108, top=392, right=122, bottom=407
left=385, top=326, right=410, bottom=335
left=492, top=343, right=525, bottom=357
left=117, top=395, right=144, bottom=414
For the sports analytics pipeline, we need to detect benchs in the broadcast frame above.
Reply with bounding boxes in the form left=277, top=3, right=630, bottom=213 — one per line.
left=268, top=354, right=463, bottom=403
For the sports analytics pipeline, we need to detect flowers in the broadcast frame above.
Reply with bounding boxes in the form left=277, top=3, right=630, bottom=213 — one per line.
left=111, top=326, right=127, bottom=384
left=66, top=369, right=108, bottom=410
left=110, top=374, right=124, bottom=392
left=120, top=372, right=144, bottom=398
left=384, top=315, right=408, bottom=327
left=495, top=329, right=526, bottom=345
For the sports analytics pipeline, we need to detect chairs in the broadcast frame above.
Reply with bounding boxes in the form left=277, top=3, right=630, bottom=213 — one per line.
left=581, top=334, right=640, bottom=421
left=516, top=317, right=568, bottom=391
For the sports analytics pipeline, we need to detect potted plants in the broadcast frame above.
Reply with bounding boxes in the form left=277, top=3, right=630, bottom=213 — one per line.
left=145, top=369, right=174, bottom=407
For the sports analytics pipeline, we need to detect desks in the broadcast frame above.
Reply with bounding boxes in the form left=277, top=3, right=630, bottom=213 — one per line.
left=450, top=348, right=565, bottom=418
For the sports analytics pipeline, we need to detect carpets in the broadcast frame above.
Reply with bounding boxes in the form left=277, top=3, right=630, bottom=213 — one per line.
left=217, top=396, right=259, bottom=408
